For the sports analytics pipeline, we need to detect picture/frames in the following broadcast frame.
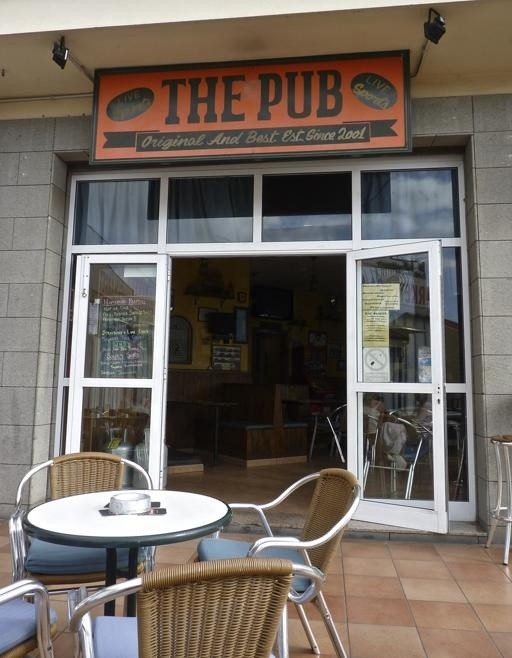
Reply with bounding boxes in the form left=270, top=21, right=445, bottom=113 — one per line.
left=210, top=343, right=241, bottom=371
left=198, top=306, right=218, bottom=322
left=232, top=306, right=249, bottom=344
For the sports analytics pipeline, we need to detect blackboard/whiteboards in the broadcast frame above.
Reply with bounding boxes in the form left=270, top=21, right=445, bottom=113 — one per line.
left=97, top=295, right=156, bottom=378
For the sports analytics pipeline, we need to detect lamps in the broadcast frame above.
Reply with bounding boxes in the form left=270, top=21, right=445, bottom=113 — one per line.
left=51, top=36, right=70, bottom=70
left=423, top=8, right=446, bottom=45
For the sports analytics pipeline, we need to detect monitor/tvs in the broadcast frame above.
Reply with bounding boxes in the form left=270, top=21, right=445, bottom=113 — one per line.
left=253, top=287, right=293, bottom=321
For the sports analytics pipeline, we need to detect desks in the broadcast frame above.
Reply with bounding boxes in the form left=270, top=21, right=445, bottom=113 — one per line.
left=168, top=399, right=240, bottom=466
left=280, top=399, right=343, bottom=454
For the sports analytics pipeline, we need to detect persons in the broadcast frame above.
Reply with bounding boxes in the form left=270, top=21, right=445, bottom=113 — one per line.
left=413, top=393, right=432, bottom=425
left=363, top=392, right=386, bottom=442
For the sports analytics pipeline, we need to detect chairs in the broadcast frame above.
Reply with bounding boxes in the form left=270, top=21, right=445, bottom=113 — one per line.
left=309, top=403, right=467, bottom=500
left=188, top=467, right=363, bottom=658
left=66, top=557, right=324, bottom=658
left=8, top=451, right=157, bottom=595
left=0, top=577, right=58, bottom=657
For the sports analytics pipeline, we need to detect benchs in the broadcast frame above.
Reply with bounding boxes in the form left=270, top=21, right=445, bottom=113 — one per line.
left=278, top=384, right=309, bottom=456
left=193, top=382, right=279, bottom=461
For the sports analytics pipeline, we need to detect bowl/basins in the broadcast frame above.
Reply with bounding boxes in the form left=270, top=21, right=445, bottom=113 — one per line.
left=109, top=492, right=151, bottom=515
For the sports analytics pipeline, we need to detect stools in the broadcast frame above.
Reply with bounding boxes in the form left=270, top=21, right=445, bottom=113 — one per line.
left=484, top=434, right=512, bottom=565
